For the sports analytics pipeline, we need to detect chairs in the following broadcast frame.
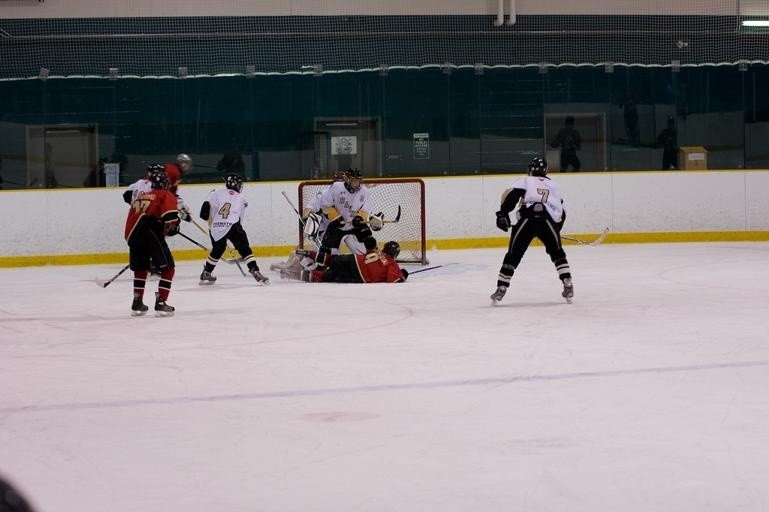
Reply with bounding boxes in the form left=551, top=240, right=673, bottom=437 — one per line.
left=200, top=271, right=216, bottom=281
left=155, top=299, right=175, bottom=312
left=254, top=272, right=268, bottom=282
left=490, top=286, right=506, bottom=301
left=562, top=284, right=573, bottom=297
left=131, top=299, right=148, bottom=311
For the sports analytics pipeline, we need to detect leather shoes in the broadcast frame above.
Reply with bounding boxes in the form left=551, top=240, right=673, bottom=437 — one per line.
left=352, top=216, right=363, bottom=226
left=332, top=216, right=345, bottom=228
left=179, top=208, right=191, bottom=221
left=496, top=211, right=511, bottom=231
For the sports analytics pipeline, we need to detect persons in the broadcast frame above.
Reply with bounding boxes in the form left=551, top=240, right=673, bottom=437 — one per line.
left=122, top=162, right=192, bottom=222
left=490, top=157, right=574, bottom=301
left=280, top=167, right=408, bottom=283
left=166, top=154, right=192, bottom=183
left=124, top=173, right=180, bottom=312
left=656, top=115, right=687, bottom=170
left=551, top=116, right=582, bottom=172
left=199, top=174, right=269, bottom=283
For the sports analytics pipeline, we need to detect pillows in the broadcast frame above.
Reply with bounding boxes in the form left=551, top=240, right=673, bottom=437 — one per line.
left=332, top=168, right=363, bottom=191
left=225, top=174, right=243, bottom=193
left=528, top=157, right=548, bottom=176
left=147, top=153, right=192, bottom=189
left=383, top=240, right=400, bottom=258
